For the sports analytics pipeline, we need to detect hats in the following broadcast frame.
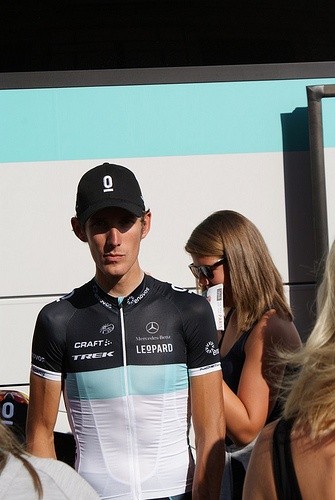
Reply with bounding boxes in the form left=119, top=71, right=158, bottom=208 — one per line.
left=76, top=163, right=145, bottom=231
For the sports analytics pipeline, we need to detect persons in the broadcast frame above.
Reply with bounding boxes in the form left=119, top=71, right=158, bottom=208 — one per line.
left=184, top=209, right=303, bottom=500
left=0, top=419, right=106, bottom=500
left=241, top=237, right=334, bottom=500
left=24, top=163, right=226, bottom=500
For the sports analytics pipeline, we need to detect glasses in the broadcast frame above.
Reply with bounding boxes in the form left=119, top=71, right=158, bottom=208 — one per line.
left=188, top=257, right=226, bottom=280
